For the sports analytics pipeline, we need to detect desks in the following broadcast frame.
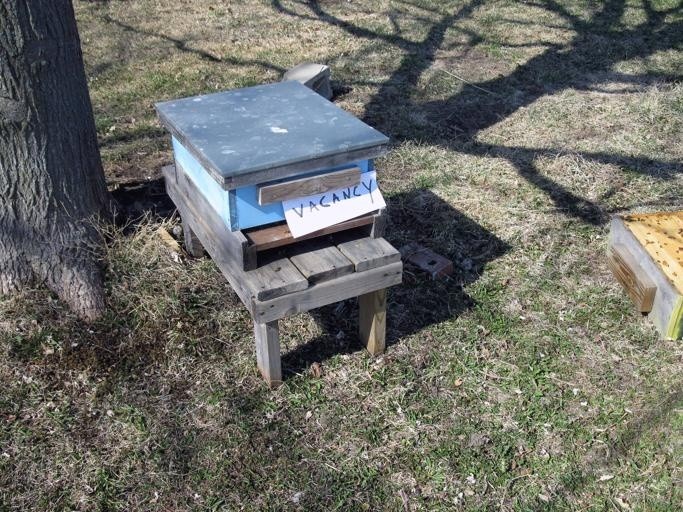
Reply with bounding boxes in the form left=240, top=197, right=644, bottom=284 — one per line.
left=162, top=166, right=403, bottom=392
